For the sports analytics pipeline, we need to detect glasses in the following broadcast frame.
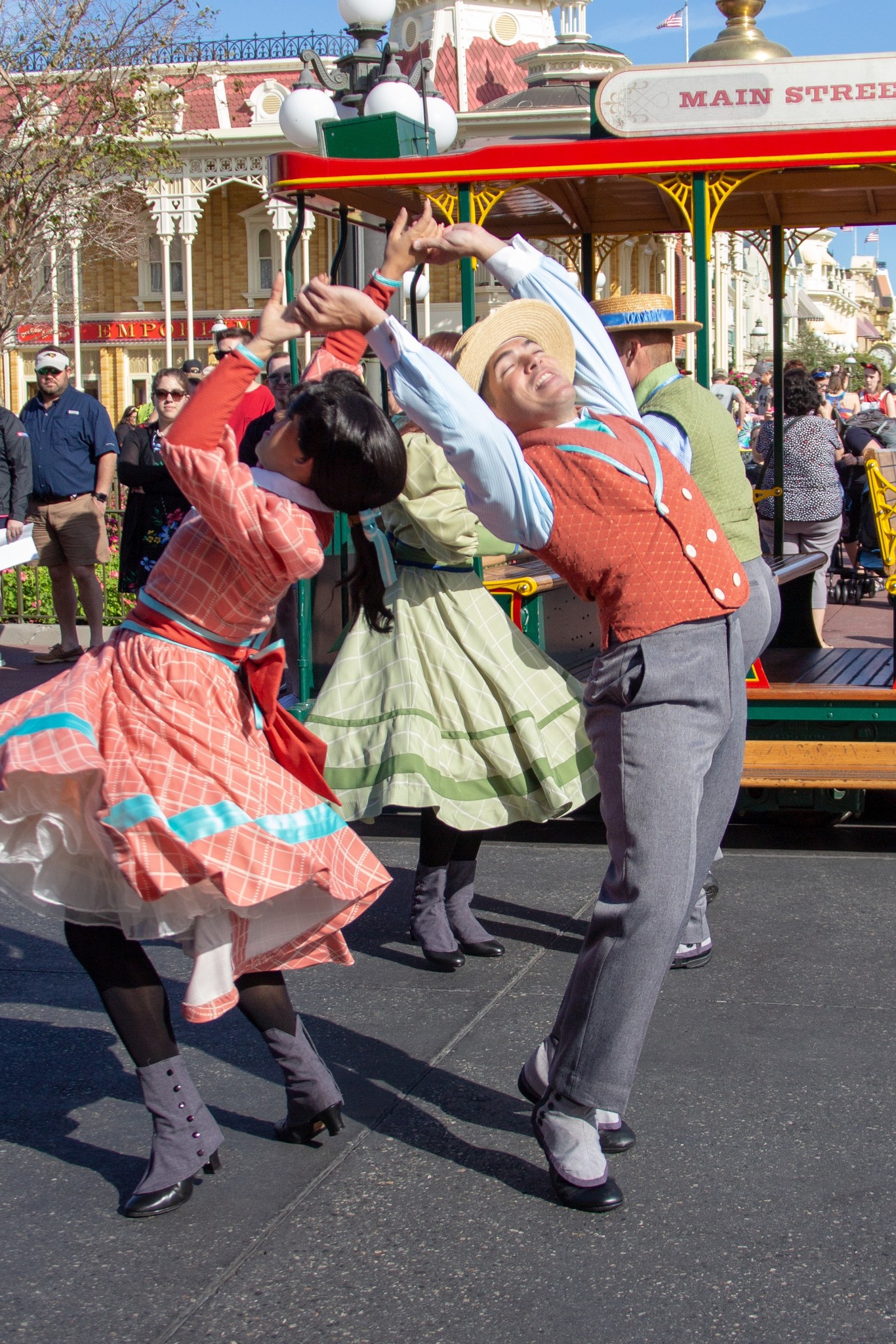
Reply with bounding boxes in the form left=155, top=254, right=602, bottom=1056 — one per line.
left=817, top=383, right=829, bottom=388
left=213, top=350, right=232, bottom=361
left=267, top=372, right=301, bottom=384
left=152, top=388, right=186, bottom=402
left=755, top=374, right=767, bottom=381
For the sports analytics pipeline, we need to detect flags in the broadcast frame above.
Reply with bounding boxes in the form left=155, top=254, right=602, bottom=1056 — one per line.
left=865, top=227, right=879, bottom=243
left=840, top=226, right=854, bottom=232
left=657, top=6, right=684, bottom=28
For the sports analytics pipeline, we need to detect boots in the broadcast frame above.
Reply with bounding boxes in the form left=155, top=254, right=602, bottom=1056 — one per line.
left=261, top=1012, right=345, bottom=1143
left=445, top=859, right=505, bottom=957
left=410, top=860, right=465, bottom=967
left=123, top=1055, right=223, bottom=1217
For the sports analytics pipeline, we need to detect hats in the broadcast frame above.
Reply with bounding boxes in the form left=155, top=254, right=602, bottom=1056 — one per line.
left=451, top=298, right=577, bottom=393
left=713, top=368, right=728, bottom=377
left=35, top=350, right=69, bottom=371
left=749, top=361, right=770, bottom=379
left=183, top=359, right=202, bottom=373
left=588, top=294, right=703, bottom=336
left=202, top=366, right=213, bottom=375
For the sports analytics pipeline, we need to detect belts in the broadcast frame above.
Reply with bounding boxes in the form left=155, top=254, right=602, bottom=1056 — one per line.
left=33, top=490, right=94, bottom=504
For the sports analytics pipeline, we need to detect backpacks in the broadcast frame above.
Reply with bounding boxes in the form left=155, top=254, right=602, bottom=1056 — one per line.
left=844, top=409, right=896, bottom=449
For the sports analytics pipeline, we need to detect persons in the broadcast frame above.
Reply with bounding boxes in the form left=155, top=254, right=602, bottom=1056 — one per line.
left=710, top=356, right=895, bottom=649
left=593, top=291, right=782, bottom=972
left=1, top=198, right=442, bottom=1219
left=301, top=224, right=749, bottom=1210
left=307, top=330, right=603, bottom=970
left=18, top=347, right=120, bottom=663
left=2, top=405, right=32, bottom=540
left=114, top=327, right=314, bottom=710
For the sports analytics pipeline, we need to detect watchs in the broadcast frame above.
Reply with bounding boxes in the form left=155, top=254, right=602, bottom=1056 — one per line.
left=93, top=492, right=108, bottom=501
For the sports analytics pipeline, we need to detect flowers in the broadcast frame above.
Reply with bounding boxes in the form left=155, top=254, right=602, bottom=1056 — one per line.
left=727, top=369, right=758, bottom=396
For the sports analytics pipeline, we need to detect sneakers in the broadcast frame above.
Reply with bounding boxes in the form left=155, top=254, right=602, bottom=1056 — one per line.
left=702, top=870, right=719, bottom=904
left=670, top=936, right=712, bottom=969
left=874, top=578, right=885, bottom=592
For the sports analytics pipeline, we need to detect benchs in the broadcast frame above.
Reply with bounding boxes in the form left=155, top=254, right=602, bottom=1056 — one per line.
left=745, top=543, right=828, bottom=692
left=473, top=552, right=603, bottom=656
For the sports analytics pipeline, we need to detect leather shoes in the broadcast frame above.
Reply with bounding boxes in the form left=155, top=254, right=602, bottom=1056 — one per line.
left=519, top=1034, right=637, bottom=1154
left=33, top=643, right=85, bottom=663
left=530, top=1084, right=624, bottom=1212
